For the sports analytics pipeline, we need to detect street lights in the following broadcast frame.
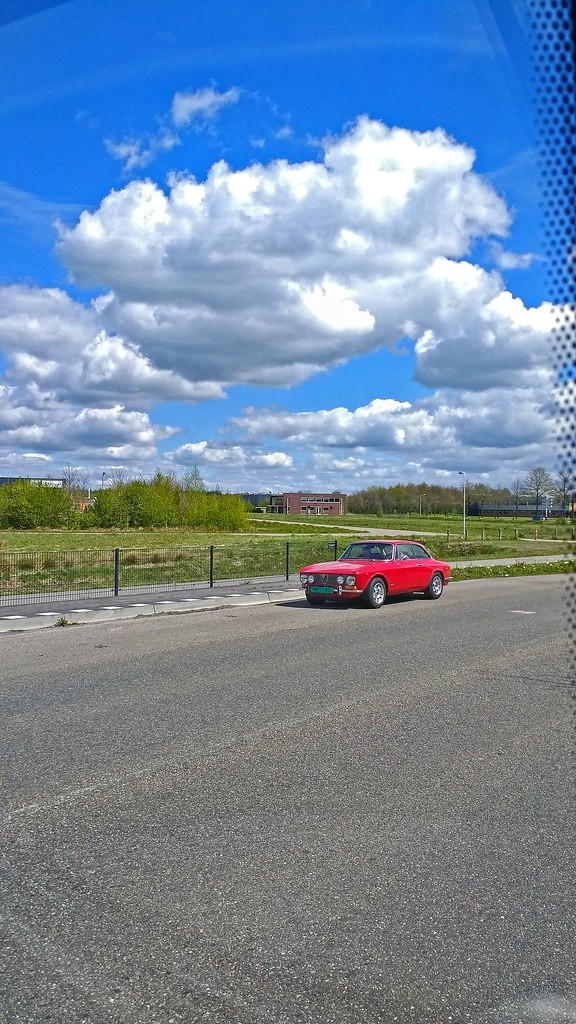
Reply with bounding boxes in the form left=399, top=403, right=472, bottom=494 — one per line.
left=420, top=493, right=426, bottom=516
left=458, top=471, right=465, bottom=533
left=546, top=490, right=555, bottom=520
left=101, top=472, right=105, bottom=491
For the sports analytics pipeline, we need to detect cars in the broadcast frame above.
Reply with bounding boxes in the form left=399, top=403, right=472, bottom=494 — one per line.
left=297, top=539, right=453, bottom=607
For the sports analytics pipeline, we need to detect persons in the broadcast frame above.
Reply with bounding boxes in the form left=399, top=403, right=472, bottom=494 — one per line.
left=384, top=551, right=410, bottom=559
left=360, top=546, right=379, bottom=559
